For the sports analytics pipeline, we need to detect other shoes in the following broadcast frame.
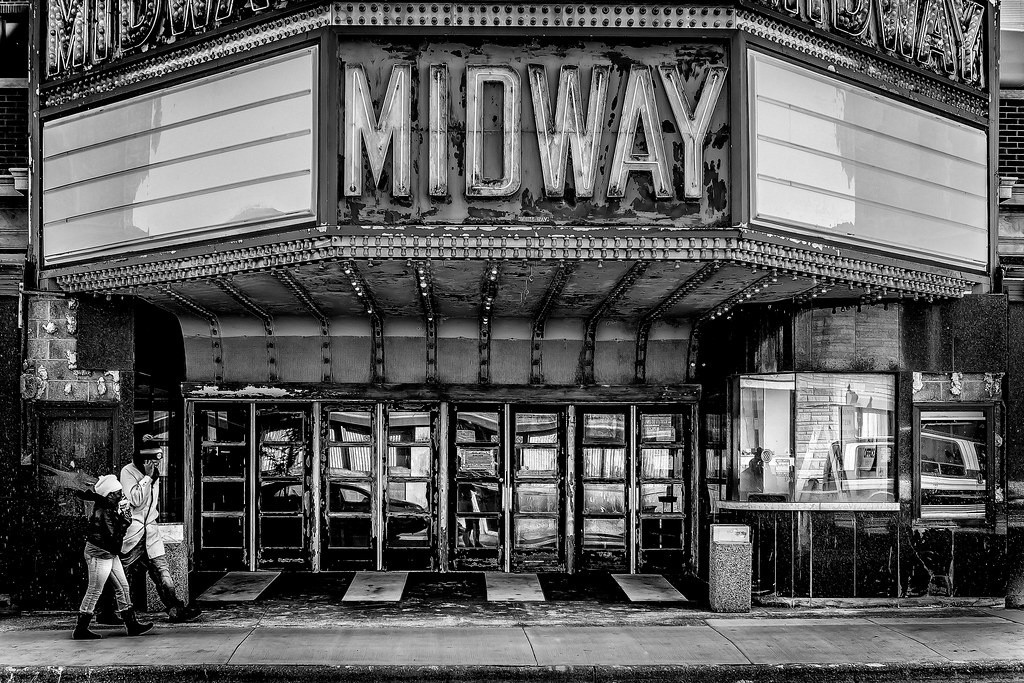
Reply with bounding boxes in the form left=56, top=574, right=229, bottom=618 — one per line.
left=162, top=601, right=204, bottom=623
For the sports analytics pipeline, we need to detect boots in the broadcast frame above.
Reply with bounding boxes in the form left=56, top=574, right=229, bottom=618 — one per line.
left=71, top=612, right=102, bottom=639
left=115, top=606, right=153, bottom=636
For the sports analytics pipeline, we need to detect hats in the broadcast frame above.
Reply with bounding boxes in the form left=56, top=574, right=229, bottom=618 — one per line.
left=94, top=474, right=123, bottom=497
left=139, top=434, right=163, bottom=460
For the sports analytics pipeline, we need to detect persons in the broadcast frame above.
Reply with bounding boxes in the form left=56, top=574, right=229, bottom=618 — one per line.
left=96, top=434, right=202, bottom=625
left=72, top=474, right=154, bottom=641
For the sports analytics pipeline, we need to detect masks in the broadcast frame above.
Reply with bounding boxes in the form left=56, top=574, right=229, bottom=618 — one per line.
left=107, top=491, right=122, bottom=503
